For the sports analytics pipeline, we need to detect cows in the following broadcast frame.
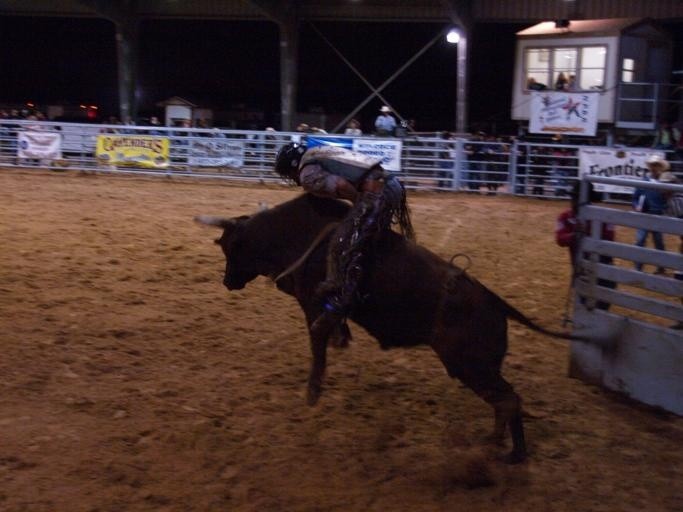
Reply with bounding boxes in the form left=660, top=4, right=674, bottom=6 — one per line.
left=191, top=191, right=630, bottom=468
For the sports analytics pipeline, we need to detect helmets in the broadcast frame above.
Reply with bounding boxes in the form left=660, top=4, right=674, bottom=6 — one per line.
left=274, top=142, right=307, bottom=186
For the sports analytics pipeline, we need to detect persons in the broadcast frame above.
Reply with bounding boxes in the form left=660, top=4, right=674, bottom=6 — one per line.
left=101, top=117, right=226, bottom=162
left=631, top=154, right=677, bottom=274
left=344, top=119, right=362, bottom=136
left=438, top=122, right=683, bottom=202
left=525, top=72, right=576, bottom=93
left=2, top=109, right=48, bottom=166
left=556, top=182, right=618, bottom=312
left=275, top=142, right=403, bottom=315
left=374, top=105, right=397, bottom=136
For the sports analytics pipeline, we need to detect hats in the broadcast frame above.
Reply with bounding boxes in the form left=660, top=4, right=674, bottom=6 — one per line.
left=379, top=106, right=390, bottom=112
left=644, top=155, right=668, bottom=171
left=346, top=119, right=360, bottom=128
left=563, top=181, right=602, bottom=202
